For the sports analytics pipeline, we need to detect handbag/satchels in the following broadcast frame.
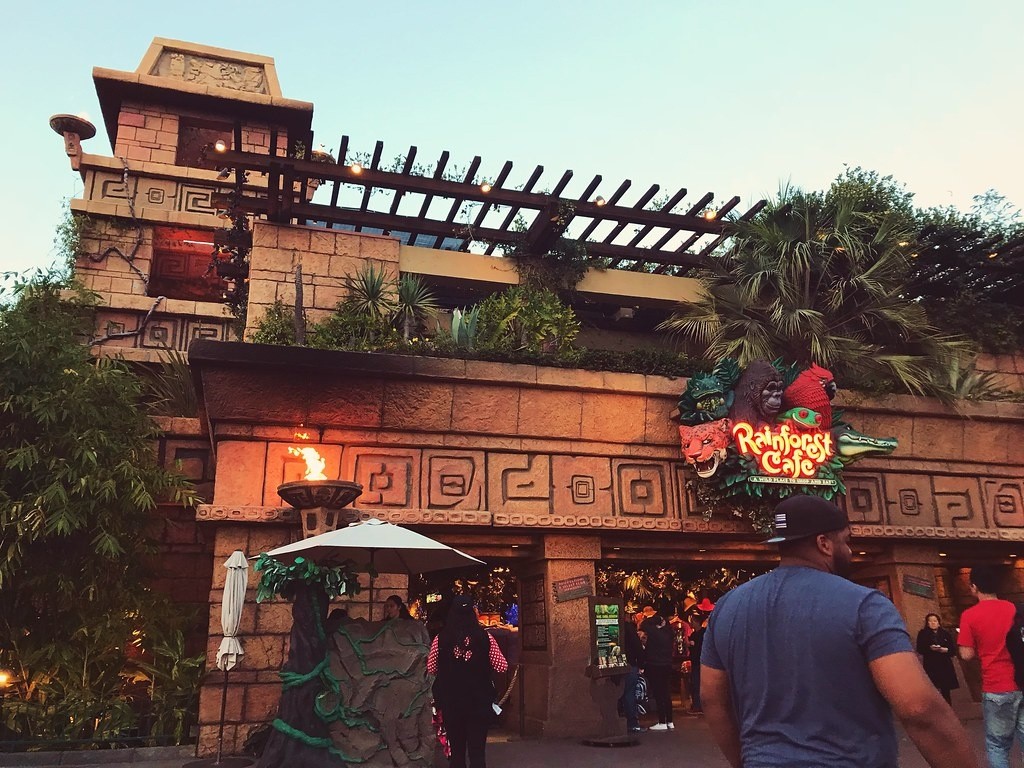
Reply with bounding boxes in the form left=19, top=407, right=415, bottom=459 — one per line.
left=630, top=649, right=647, bottom=673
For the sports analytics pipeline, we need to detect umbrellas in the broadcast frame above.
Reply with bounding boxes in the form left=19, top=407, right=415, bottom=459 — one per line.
left=214, top=547, right=254, bottom=763
left=246, top=518, right=488, bottom=619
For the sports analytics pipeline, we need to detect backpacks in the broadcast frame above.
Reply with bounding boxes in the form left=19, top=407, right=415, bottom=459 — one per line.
left=635, top=672, right=649, bottom=715
left=1007, top=601, right=1024, bottom=694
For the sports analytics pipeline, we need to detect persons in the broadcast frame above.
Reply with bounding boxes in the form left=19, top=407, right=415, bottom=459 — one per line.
left=916, top=613, right=959, bottom=709
left=621, top=596, right=715, bottom=736
left=956, top=564, right=1024, bottom=768
left=698, top=495, right=979, bottom=768
left=385, top=595, right=414, bottom=621
left=427, top=595, right=507, bottom=768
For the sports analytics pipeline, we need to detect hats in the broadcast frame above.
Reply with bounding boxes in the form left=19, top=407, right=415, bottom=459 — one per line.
left=760, top=493, right=849, bottom=544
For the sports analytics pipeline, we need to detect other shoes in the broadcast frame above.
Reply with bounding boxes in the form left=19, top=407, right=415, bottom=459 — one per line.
left=686, top=706, right=703, bottom=715
left=628, top=727, right=647, bottom=733
left=650, top=722, right=674, bottom=730
